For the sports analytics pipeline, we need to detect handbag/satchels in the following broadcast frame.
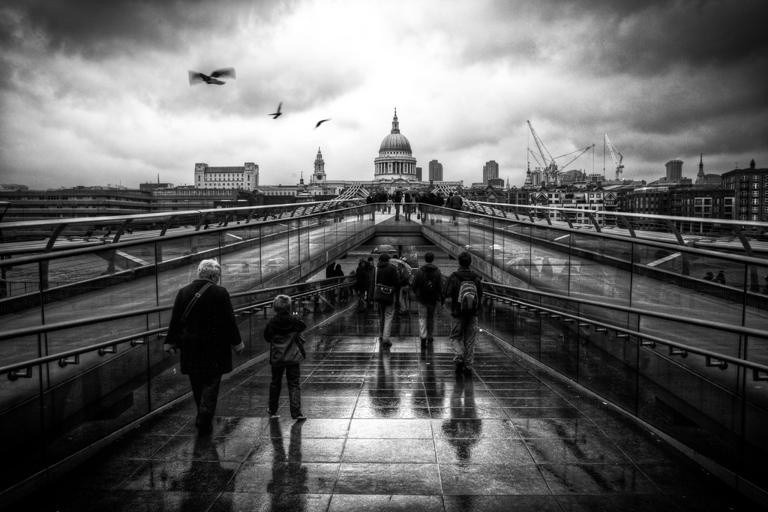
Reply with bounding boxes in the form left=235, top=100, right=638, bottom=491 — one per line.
left=373, top=283, right=395, bottom=306
left=269, top=336, right=306, bottom=364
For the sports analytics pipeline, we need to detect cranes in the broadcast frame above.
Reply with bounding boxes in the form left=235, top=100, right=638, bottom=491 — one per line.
left=527, top=120, right=624, bottom=187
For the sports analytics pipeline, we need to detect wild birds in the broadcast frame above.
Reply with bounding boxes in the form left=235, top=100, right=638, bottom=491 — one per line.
left=316, top=118, right=329, bottom=127
left=268, top=102, right=283, bottom=119
left=188, top=67, right=236, bottom=86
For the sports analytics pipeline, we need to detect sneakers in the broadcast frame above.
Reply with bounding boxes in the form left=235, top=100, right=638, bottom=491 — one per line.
left=452, top=355, right=473, bottom=366
left=379, top=337, right=393, bottom=348
left=419, top=336, right=434, bottom=347
left=264, top=407, right=308, bottom=419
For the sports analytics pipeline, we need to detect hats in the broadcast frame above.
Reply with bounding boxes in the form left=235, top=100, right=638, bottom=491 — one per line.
left=458, top=251, right=472, bottom=266
left=424, top=252, right=434, bottom=263
left=272, top=294, right=292, bottom=311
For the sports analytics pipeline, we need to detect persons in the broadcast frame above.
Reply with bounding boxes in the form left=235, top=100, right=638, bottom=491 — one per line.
left=413, top=252, right=447, bottom=350
left=163, top=258, right=245, bottom=438
left=441, top=251, right=483, bottom=371
left=366, top=185, right=464, bottom=226
left=263, top=294, right=308, bottom=419
left=325, top=255, right=414, bottom=347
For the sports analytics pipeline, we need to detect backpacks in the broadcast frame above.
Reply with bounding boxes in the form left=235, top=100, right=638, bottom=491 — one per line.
left=453, top=270, right=481, bottom=315
